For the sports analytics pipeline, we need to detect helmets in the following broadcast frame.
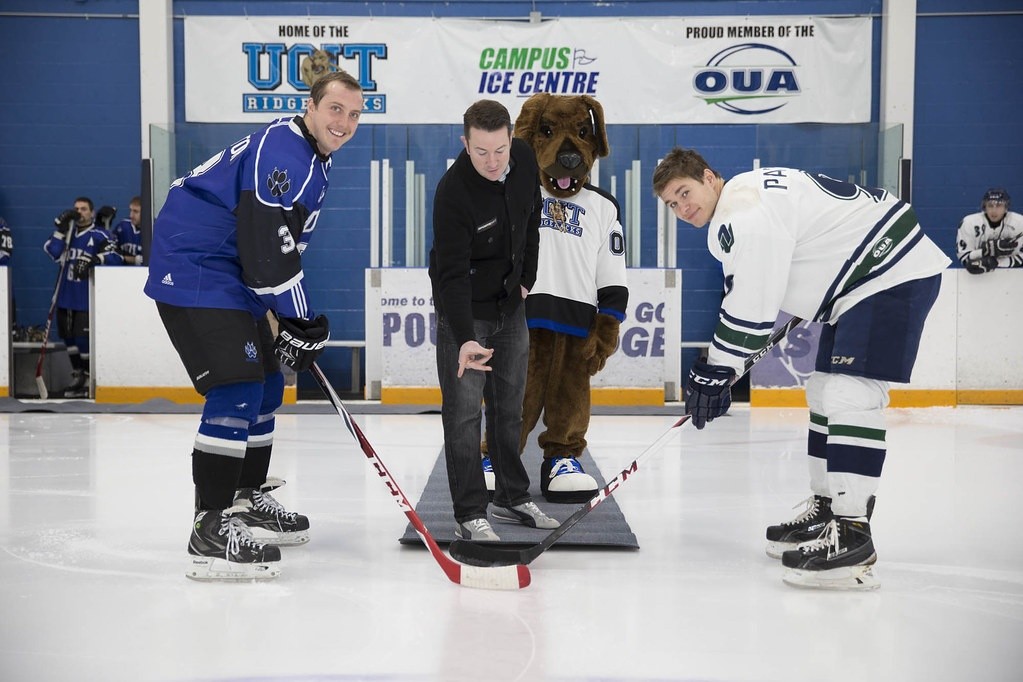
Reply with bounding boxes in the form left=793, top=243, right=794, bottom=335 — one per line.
left=982, top=187, right=1010, bottom=211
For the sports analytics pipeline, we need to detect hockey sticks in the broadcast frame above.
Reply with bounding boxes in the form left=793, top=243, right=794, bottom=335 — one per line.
left=35, top=217, right=77, bottom=400
left=310, top=358, right=533, bottom=591
left=449, top=312, right=808, bottom=568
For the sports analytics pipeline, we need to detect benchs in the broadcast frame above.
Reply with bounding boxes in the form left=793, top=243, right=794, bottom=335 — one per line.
left=324, top=340, right=366, bottom=395
left=13, top=341, right=65, bottom=356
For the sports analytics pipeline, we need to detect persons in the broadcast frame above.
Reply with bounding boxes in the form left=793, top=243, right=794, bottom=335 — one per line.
left=955, top=186, right=1023, bottom=276
left=428, top=100, right=559, bottom=539
left=1, top=197, right=144, bottom=398
left=144, top=72, right=364, bottom=582
left=651, top=147, right=953, bottom=590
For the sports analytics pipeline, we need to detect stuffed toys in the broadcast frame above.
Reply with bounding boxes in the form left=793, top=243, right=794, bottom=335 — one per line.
left=478, top=93, right=630, bottom=502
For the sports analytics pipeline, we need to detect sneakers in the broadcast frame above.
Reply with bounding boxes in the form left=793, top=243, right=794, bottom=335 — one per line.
left=64, top=370, right=85, bottom=397
left=764, top=494, right=833, bottom=560
left=84, top=371, right=90, bottom=397
left=454, top=518, right=500, bottom=541
left=184, top=498, right=282, bottom=581
left=232, top=477, right=311, bottom=547
left=491, top=502, right=560, bottom=528
left=783, top=495, right=882, bottom=590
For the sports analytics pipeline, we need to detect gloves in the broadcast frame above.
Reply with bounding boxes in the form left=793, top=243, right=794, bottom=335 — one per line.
left=685, top=357, right=732, bottom=429
left=966, top=256, right=998, bottom=274
left=982, top=238, right=1018, bottom=256
left=83, top=254, right=101, bottom=277
left=95, top=205, right=116, bottom=228
left=273, top=316, right=329, bottom=371
left=55, top=209, right=81, bottom=231
